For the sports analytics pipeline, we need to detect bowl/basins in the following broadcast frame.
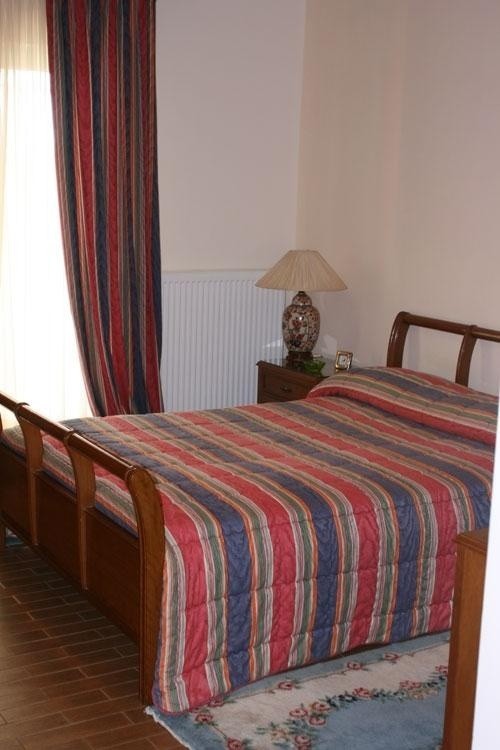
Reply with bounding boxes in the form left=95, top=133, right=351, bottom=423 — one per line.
left=303, top=360, right=326, bottom=373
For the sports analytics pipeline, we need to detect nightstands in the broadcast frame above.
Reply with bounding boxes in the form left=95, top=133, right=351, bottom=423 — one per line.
left=257, top=354, right=354, bottom=403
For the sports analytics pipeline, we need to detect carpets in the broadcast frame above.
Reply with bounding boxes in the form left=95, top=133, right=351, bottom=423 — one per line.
left=145, top=628, right=450, bottom=750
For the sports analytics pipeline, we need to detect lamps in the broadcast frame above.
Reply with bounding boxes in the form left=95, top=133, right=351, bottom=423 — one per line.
left=254, top=248, right=348, bottom=363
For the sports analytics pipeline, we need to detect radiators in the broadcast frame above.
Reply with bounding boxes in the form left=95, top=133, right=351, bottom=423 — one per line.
left=160, top=269, right=286, bottom=414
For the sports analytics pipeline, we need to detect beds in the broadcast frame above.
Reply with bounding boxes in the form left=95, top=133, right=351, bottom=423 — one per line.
left=0, top=311, right=500, bottom=716
